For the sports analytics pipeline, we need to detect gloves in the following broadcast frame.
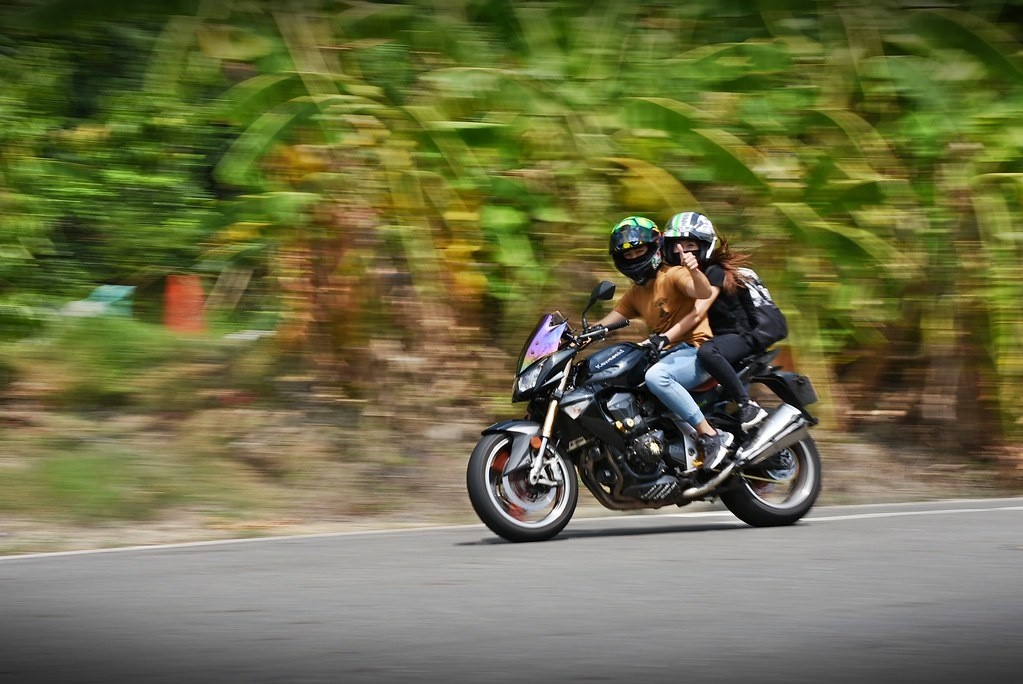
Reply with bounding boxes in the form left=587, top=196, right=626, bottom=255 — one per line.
left=637, top=332, right=671, bottom=364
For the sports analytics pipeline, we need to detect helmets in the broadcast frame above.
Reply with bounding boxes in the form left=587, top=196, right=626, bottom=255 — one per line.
left=608, top=217, right=662, bottom=285
left=661, top=212, right=718, bottom=267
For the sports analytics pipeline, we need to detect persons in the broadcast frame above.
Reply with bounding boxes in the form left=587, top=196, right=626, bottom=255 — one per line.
left=581, top=211, right=768, bottom=471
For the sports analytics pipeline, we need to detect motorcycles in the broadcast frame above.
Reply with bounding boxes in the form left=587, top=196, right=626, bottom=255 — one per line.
left=466, top=281, right=822, bottom=543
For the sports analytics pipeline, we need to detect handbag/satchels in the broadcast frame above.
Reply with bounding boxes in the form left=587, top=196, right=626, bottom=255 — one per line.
left=735, top=276, right=788, bottom=349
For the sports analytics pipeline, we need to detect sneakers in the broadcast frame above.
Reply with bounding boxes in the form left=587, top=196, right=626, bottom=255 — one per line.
left=689, top=428, right=734, bottom=470
left=737, top=401, right=769, bottom=431
